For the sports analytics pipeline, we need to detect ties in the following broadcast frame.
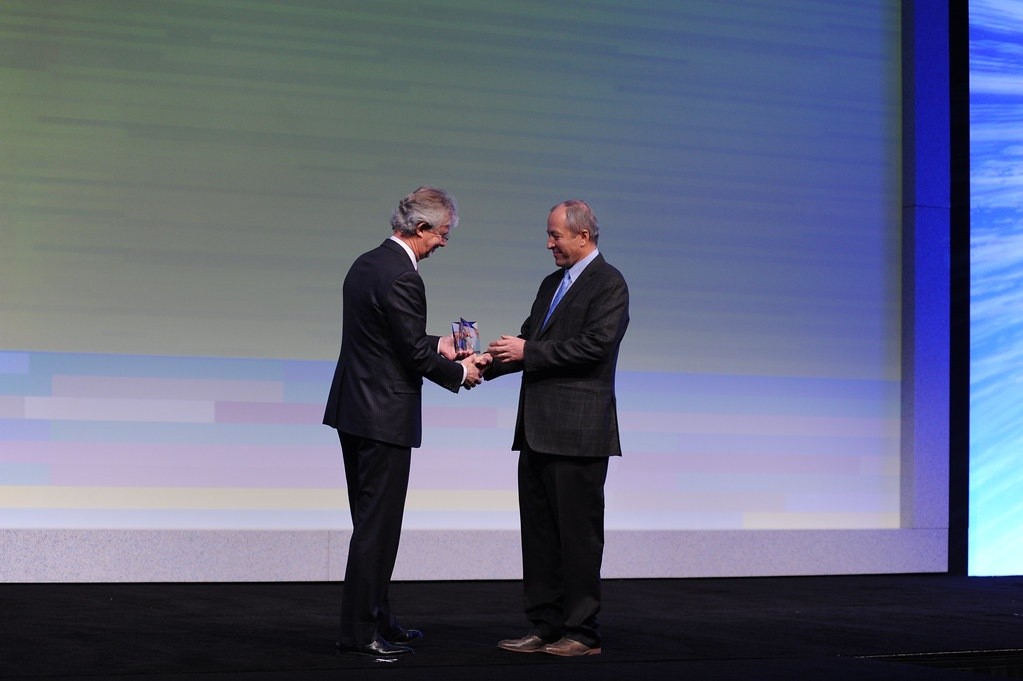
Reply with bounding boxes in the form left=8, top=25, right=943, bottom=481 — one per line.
left=541, top=271, right=569, bottom=332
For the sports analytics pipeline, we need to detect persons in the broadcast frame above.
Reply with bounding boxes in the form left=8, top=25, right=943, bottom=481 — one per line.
left=322, top=184, right=482, bottom=665
left=472, top=200, right=630, bottom=657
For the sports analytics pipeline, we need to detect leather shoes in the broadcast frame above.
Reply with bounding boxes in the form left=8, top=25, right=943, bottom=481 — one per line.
left=336, top=635, right=414, bottom=662
left=541, top=637, right=602, bottom=656
left=379, top=623, right=423, bottom=645
left=497, top=633, right=553, bottom=652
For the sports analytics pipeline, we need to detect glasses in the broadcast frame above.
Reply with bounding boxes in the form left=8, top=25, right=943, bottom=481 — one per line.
left=432, top=227, right=448, bottom=243
